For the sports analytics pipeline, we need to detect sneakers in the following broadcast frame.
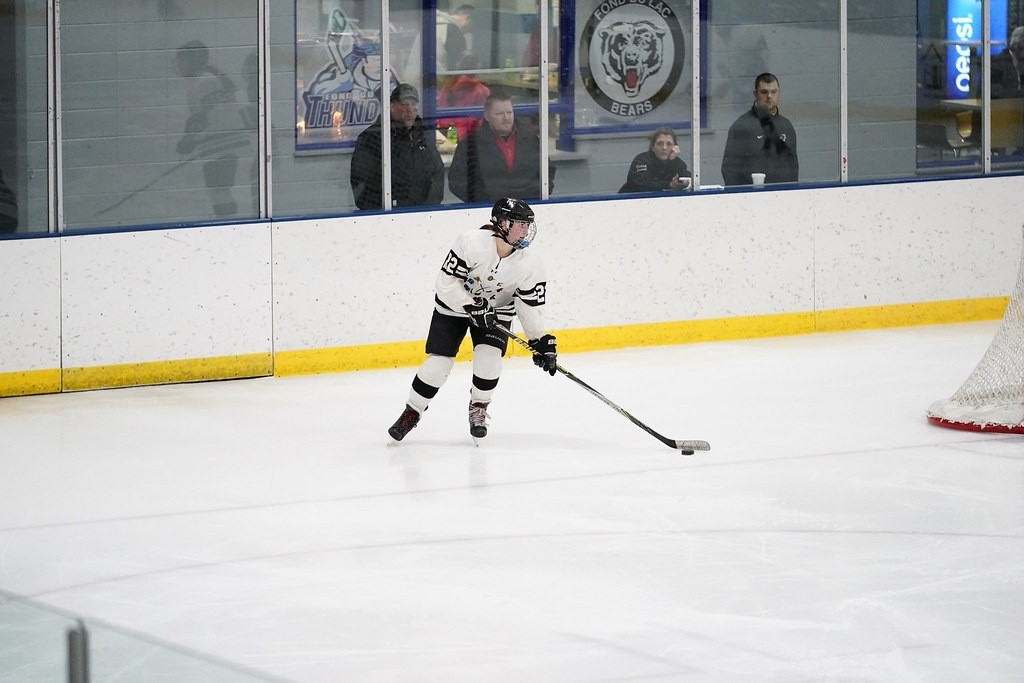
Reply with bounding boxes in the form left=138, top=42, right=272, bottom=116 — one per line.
left=386, top=401, right=428, bottom=447
left=469, top=388, right=491, bottom=447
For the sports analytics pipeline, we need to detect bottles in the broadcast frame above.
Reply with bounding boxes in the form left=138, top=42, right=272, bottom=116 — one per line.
left=447, top=122, right=458, bottom=147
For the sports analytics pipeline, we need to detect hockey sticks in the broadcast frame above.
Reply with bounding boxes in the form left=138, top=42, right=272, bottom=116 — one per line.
left=491, top=319, right=712, bottom=451
left=92, top=159, right=188, bottom=219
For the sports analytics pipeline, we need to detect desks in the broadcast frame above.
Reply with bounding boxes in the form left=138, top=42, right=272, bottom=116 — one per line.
left=943, top=98, right=1024, bottom=161
left=476, top=73, right=559, bottom=101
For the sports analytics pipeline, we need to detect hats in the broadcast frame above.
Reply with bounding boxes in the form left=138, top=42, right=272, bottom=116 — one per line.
left=390, top=83, right=419, bottom=102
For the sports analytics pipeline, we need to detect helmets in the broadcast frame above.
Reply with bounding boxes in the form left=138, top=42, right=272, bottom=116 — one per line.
left=491, top=198, right=537, bottom=250
left=172, top=40, right=209, bottom=76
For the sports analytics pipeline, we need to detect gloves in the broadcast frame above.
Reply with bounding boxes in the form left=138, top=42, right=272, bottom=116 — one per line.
left=528, top=334, right=557, bottom=376
left=462, top=298, right=498, bottom=330
left=176, top=134, right=199, bottom=154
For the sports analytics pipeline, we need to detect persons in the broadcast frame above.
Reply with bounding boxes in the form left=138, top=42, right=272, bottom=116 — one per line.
left=350, top=84, right=445, bottom=210
left=171, top=39, right=250, bottom=216
left=404, top=4, right=475, bottom=113
left=721, top=73, right=800, bottom=185
left=390, top=197, right=557, bottom=442
left=448, top=93, right=556, bottom=201
left=970, top=27, right=1024, bottom=160
left=618, top=127, right=692, bottom=192
left=435, top=49, right=494, bottom=142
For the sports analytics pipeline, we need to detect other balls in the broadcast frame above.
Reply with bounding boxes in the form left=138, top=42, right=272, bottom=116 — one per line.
left=681, top=449, right=694, bottom=455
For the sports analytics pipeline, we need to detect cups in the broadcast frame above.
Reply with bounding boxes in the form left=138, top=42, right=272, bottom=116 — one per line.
left=679, top=176, right=692, bottom=190
left=751, top=173, right=766, bottom=185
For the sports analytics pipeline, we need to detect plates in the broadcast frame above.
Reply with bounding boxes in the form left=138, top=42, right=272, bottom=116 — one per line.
left=436, top=140, right=445, bottom=143
